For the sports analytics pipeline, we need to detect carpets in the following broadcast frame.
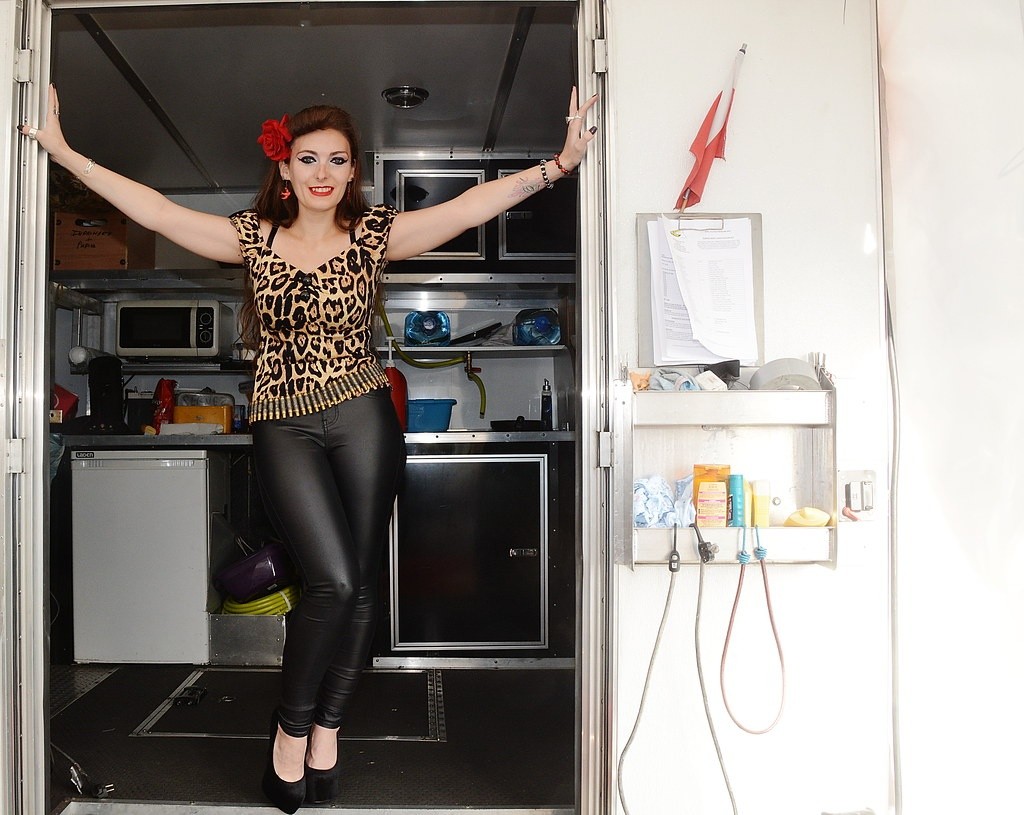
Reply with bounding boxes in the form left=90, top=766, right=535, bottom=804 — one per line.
left=50, top=665, right=580, bottom=809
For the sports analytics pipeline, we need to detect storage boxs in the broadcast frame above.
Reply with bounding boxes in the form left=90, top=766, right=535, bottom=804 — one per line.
left=49, top=382, right=80, bottom=423
left=52, top=207, right=154, bottom=272
left=209, top=607, right=286, bottom=669
left=174, top=393, right=235, bottom=434
left=407, top=398, right=458, bottom=433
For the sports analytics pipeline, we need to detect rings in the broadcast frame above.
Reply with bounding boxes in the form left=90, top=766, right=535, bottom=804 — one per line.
left=565, top=112, right=582, bottom=124
left=54, top=109, right=60, bottom=115
left=29, top=128, right=36, bottom=139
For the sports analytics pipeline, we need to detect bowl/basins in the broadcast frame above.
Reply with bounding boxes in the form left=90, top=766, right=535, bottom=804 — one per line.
left=407, top=400, right=455, bottom=432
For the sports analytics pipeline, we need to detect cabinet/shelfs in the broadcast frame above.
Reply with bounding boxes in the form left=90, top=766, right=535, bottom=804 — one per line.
left=373, top=152, right=576, bottom=274
left=628, top=386, right=832, bottom=565
left=389, top=454, right=560, bottom=651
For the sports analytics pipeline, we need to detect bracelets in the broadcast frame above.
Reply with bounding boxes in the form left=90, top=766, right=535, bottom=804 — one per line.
left=554, top=153, right=573, bottom=177
left=541, top=159, right=554, bottom=190
left=76, top=158, right=95, bottom=180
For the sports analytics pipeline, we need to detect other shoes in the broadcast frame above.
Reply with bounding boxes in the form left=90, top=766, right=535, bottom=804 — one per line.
left=262, top=707, right=306, bottom=815
left=305, top=713, right=341, bottom=803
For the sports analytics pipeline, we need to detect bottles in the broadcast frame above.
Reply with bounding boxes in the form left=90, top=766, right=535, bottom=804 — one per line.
left=404, top=311, right=451, bottom=345
left=513, top=308, right=562, bottom=345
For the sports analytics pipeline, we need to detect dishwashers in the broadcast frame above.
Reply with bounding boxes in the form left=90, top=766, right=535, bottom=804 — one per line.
left=71, top=449, right=211, bottom=665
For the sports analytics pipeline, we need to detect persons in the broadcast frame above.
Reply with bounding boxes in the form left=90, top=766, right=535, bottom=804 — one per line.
left=17, top=84, right=599, bottom=815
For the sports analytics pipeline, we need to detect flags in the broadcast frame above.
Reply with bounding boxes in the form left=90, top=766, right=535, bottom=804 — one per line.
left=675, top=87, right=734, bottom=210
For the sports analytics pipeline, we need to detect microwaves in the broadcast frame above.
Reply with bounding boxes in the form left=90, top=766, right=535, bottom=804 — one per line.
left=116, top=300, right=234, bottom=358
